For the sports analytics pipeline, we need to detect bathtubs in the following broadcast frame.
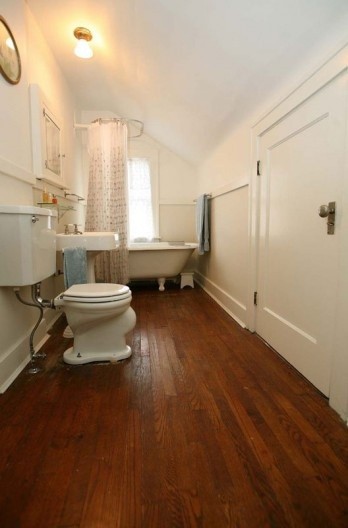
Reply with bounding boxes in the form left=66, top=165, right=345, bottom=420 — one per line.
left=128, top=240, right=199, bottom=292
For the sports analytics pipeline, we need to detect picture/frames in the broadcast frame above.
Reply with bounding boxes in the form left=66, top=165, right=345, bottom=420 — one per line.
left=1, top=13, right=21, bottom=86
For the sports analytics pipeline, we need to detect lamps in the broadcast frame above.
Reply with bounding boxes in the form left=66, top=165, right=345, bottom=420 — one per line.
left=71, top=25, right=95, bottom=60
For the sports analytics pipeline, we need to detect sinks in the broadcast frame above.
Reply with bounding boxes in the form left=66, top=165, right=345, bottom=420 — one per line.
left=57, top=232, right=119, bottom=250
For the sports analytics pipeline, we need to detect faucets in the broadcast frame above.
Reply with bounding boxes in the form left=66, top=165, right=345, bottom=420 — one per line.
left=72, top=223, right=82, bottom=236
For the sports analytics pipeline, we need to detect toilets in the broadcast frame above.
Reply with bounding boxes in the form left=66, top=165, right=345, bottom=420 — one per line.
left=1, top=203, right=138, bottom=366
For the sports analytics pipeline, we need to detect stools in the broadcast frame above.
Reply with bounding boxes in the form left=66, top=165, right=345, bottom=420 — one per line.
left=180, top=272, right=195, bottom=289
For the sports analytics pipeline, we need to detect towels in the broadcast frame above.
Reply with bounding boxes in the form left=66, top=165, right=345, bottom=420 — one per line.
left=63, top=246, right=88, bottom=291
left=195, top=193, right=211, bottom=256
